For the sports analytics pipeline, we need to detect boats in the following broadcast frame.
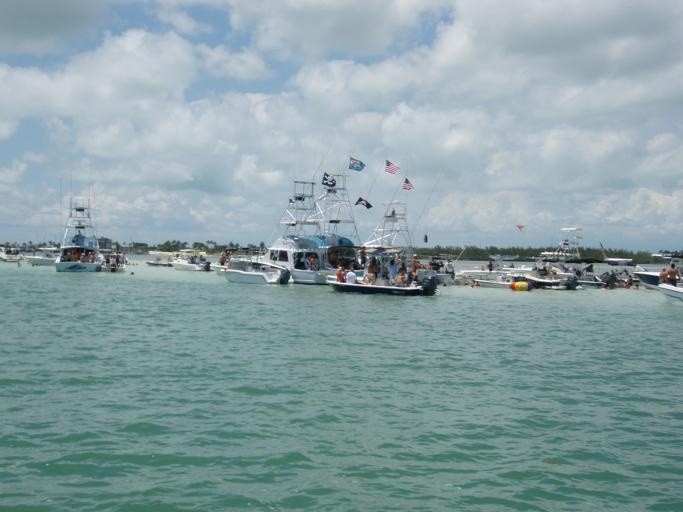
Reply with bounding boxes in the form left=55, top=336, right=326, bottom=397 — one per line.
left=0, top=197, right=129, bottom=273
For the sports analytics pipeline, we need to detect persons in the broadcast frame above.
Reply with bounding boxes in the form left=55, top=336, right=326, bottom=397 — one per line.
left=625, top=274, right=632, bottom=288
left=369, top=252, right=454, bottom=288
left=64, top=247, right=129, bottom=270
left=667, top=263, right=680, bottom=287
left=623, top=282, right=628, bottom=289
left=486, top=260, right=494, bottom=272
left=335, top=266, right=345, bottom=283
left=659, top=268, right=668, bottom=283
left=344, top=267, right=357, bottom=283
left=359, top=246, right=366, bottom=267
left=308, top=253, right=318, bottom=270
left=219, top=252, right=225, bottom=266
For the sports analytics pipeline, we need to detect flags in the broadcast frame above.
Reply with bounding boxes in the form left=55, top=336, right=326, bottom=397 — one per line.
left=401, top=179, right=414, bottom=191
left=321, top=174, right=337, bottom=187
left=349, top=157, right=365, bottom=172
left=384, top=160, right=400, bottom=176
left=355, top=197, right=372, bottom=210
